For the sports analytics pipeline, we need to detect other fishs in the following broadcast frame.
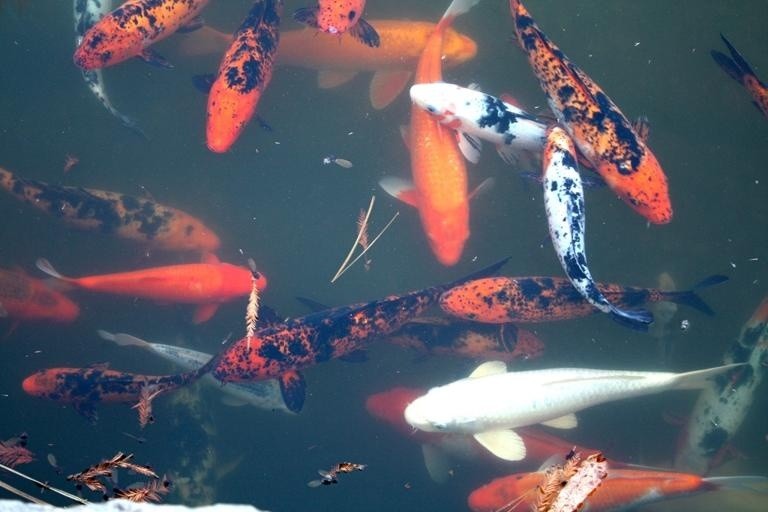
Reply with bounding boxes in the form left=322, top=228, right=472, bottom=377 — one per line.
left=0, top=1, right=768, bottom=511
